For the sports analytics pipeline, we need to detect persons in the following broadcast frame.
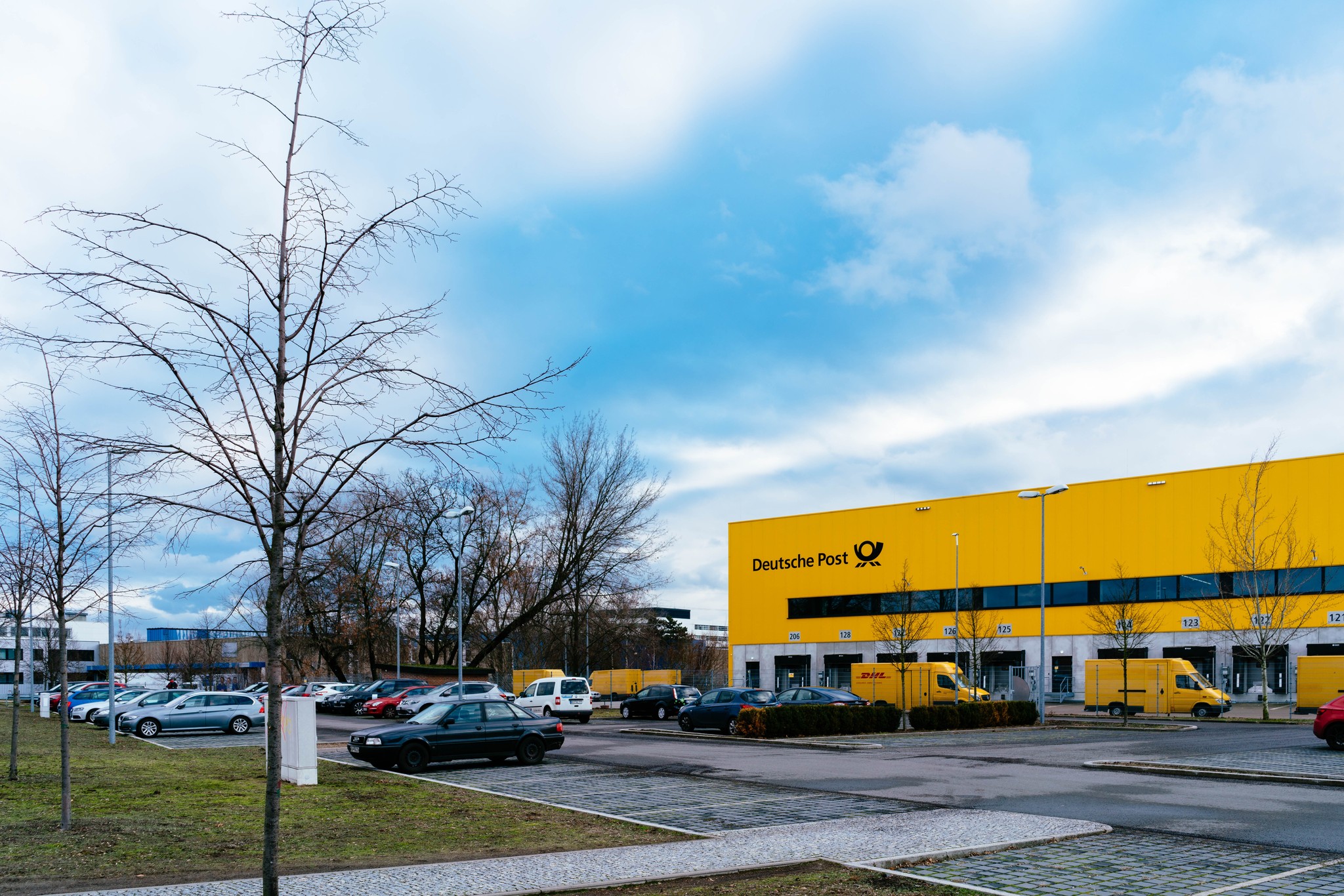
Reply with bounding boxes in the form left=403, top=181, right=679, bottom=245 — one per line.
left=232, top=677, right=240, bottom=691
left=166, top=679, right=178, bottom=689
left=226, top=677, right=233, bottom=692
left=217, top=677, right=227, bottom=692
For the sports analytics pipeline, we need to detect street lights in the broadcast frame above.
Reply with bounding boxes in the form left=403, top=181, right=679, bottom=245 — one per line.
left=1022, top=484, right=1069, bottom=725
left=26, top=565, right=34, bottom=712
left=447, top=506, right=474, bottom=701
left=951, top=533, right=960, bottom=705
left=384, top=561, right=400, bottom=678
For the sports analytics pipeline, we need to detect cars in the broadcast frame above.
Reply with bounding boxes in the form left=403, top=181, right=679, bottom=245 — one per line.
left=33, top=681, right=265, bottom=738
left=363, top=681, right=516, bottom=720
left=230, top=682, right=300, bottom=705
left=678, top=687, right=782, bottom=736
left=774, top=687, right=870, bottom=707
left=1313, top=695, right=1344, bottom=751
left=347, top=699, right=565, bottom=774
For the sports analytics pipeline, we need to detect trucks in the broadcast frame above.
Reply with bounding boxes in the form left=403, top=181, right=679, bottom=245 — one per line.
left=512, top=669, right=566, bottom=699
left=1290, top=656, right=1344, bottom=715
left=851, top=662, right=990, bottom=711
left=589, top=669, right=681, bottom=701
left=1081, top=658, right=1232, bottom=717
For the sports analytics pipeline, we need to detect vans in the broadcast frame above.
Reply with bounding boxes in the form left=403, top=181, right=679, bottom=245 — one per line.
left=513, top=677, right=593, bottom=724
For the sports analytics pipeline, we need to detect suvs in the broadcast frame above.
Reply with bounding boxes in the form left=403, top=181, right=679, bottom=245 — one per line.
left=296, top=682, right=355, bottom=707
left=321, top=678, right=429, bottom=716
left=620, top=684, right=702, bottom=722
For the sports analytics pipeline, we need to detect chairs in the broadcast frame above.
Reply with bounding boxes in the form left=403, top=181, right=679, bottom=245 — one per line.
left=460, top=709, right=481, bottom=722
left=801, top=692, right=810, bottom=700
left=540, top=687, right=554, bottom=695
left=721, top=692, right=731, bottom=703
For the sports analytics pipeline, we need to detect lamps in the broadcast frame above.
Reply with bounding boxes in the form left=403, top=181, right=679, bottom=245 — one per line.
left=1079, top=567, right=1087, bottom=575
left=1311, top=550, right=1317, bottom=560
left=902, top=579, right=910, bottom=586
